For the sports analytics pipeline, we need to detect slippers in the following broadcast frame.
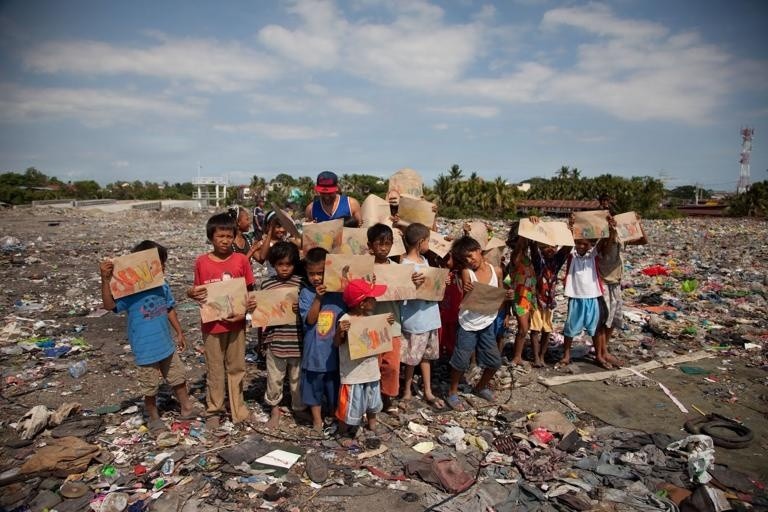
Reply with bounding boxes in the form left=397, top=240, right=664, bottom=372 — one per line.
left=309, top=426, right=323, bottom=440
left=335, top=431, right=353, bottom=448
left=365, top=423, right=391, bottom=442
left=558, top=360, right=572, bottom=369
left=427, top=394, right=468, bottom=410
left=149, top=417, right=168, bottom=437
left=471, top=387, right=498, bottom=402
left=175, top=406, right=206, bottom=421
left=398, top=395, right=414, bottom=410
left=597, top=355, right=624, bottom=368
left=387, top=400, right=399, bottom=417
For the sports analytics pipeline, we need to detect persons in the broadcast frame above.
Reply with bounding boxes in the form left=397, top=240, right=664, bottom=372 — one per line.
left=397, top=224, right=452, bottom=411
left=99, top=240, right=205, bottom=439
left=446, top=216, right=650, bottom=368
left=227, top=169, right=439, bottom=276
left=444, top=237, right=513, bottom=412
left=186, top=214, right=254, bottom=423
left=333, top=279, right=394, bottom=444
left=363, top=222, right=426, bottom=421
left=299, top=246, right=350, bottom=439
left=245, top=241, right=311, bottom=432
left=432, top=235, right=467, bottom=380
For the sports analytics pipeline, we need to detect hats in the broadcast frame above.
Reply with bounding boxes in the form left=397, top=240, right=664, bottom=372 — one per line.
left=343, top=279, right=387, bottom=306
left=315, top=171, right=339, bottom=194
